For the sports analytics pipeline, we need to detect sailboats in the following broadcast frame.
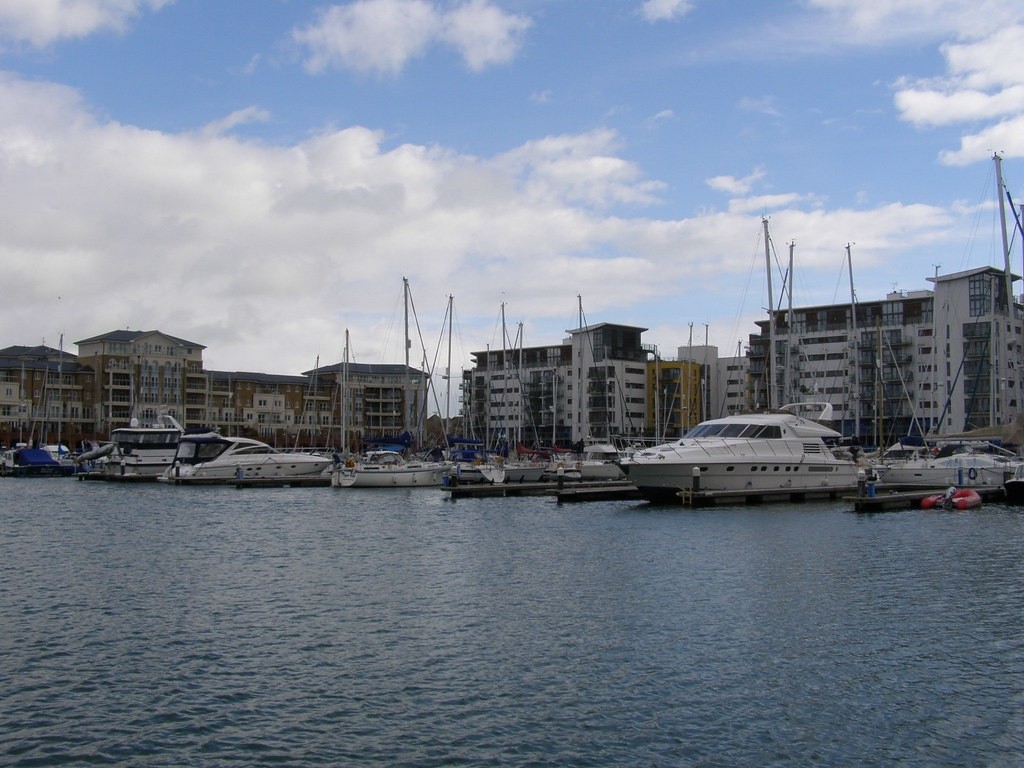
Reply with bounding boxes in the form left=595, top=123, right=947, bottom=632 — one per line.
left=0, top=332, right=108, bottom=476
left=331, top=275, right=455, bottom=488
left=448, top=293, right=633, bottom=483
left=829, top=275, right=1024, bottom=488
left=612, top=213, right=860, bottom=496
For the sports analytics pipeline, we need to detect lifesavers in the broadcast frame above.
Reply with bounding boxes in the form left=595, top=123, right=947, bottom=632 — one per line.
left=968, top=467, right=977, bottom=480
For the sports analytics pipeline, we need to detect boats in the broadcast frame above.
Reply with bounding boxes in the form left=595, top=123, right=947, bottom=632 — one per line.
left=921, top=489, right=982, bottom=510
left=157, top=431, right=334, bottom=486
left=76, top=427, right=222, bottom=481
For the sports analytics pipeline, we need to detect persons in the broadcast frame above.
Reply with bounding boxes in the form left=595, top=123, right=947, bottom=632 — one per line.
left=120, top=458, right=125, bottom=475
left=932, top=444, right=938, bottom=459
left=175, top=459, right=180, bottom=477
left=499, top=433, right=506, bottom=457
left=576, top=438, right=585, bottom=454
left=84, top=439, right=99, bottom=452
